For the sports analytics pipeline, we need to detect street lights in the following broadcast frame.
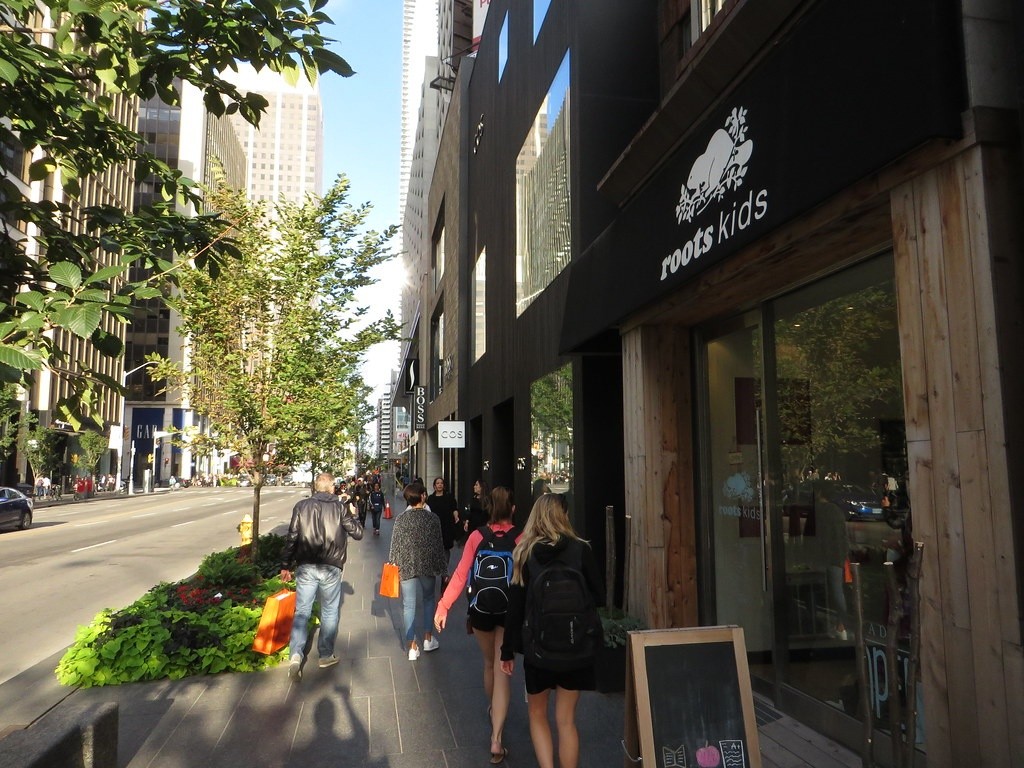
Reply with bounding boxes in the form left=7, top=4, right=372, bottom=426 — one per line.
left=114, top=361, right=158, bottom=491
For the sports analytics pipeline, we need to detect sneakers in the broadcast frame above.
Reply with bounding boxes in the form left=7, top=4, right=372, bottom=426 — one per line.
left=319, top=654, right=340, bottom=668
left=288, top=654, right=302, bottom=682
left=423, top=634, right=439, bottom=651
left=408, top=644, right=420, bottom=661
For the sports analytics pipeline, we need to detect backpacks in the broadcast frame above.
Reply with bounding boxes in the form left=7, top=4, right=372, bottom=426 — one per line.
left=521, top=535, right=599, bottom=672
left=467, top=525, right=524, bottom=622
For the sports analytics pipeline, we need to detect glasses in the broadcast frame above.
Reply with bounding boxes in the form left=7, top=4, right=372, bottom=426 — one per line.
left=341, top=485, right=346, bottom=487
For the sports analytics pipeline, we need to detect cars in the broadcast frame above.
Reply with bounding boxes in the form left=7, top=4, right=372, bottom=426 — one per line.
left=0, top=485, right=33, bottom=533
left=263, top=474, right=277, bottom=486
left=281, top=476, right=294, bottom=486
left=236, top=475, right=254, bottom=487
left=822, top=480, right=886, bottom=522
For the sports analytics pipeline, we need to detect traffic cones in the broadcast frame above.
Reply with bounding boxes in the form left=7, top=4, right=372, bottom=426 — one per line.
left=382, top=499, right=393, bottom=519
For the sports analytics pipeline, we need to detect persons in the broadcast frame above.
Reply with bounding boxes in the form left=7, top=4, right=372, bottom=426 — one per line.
left=36, top=475, right=44, bottom=500
left=191, top=474, right=218, bottom=487
left=280, top=472, right=363, bottom=683
left=109, top=476, right=114, bottom=491
left=404, top=488, right=432, bottom=512
left=42, top=475, right=50, bottom=499
left=531, top=478, right=552, bottom=506
left=427, top=477, right=459, bottom=583
left=399, top=473, right=404, bottom=491
left=881, top=470, right=914, bottom=574
left=396, top=467, right=401, bottom=478
left=389, top=481, right=448, bottom=661
left=403, top=474, right=410, bottom=489
left=369, top=482, right=386, bottom=534
left=101, top=473, right=106, bottom=490
left=465, top=480, right=493, bottom=537
left=499, top=493, right=606, bottom=768
left=169, top=476, right=176, bottom=491
left=336, top=477, right=372, bottom=527
left=434, top=486, right=524, bottom=764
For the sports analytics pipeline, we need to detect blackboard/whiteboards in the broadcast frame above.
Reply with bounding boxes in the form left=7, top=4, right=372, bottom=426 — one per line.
left=627, top=627, right=764, bottom=768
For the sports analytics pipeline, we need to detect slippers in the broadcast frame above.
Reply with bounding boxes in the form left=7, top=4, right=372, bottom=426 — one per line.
left=488, top=706, right=492, bottom=725
left=490, top=747, right=507, bottom=764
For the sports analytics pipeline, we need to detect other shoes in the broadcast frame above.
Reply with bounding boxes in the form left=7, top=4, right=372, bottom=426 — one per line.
left=442, top=574, right=449, bottom=585
left=376, top=530, right=379, bottom=535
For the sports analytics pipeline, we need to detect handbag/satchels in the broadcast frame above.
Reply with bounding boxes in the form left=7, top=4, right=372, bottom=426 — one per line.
left=252, top=579, right=297, bottom=656
left=379, top=562, right=399, bottom=598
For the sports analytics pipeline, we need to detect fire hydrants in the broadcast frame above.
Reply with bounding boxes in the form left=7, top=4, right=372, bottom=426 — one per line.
left=236, top=514, right=253, bottom=546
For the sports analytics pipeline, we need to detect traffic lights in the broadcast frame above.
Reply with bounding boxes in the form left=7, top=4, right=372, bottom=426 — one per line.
left=156, top=438, right=160, bottom=444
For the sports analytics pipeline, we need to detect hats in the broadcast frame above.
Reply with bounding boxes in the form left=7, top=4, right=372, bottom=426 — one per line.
left=358, top=477, right=364, bottom=483
